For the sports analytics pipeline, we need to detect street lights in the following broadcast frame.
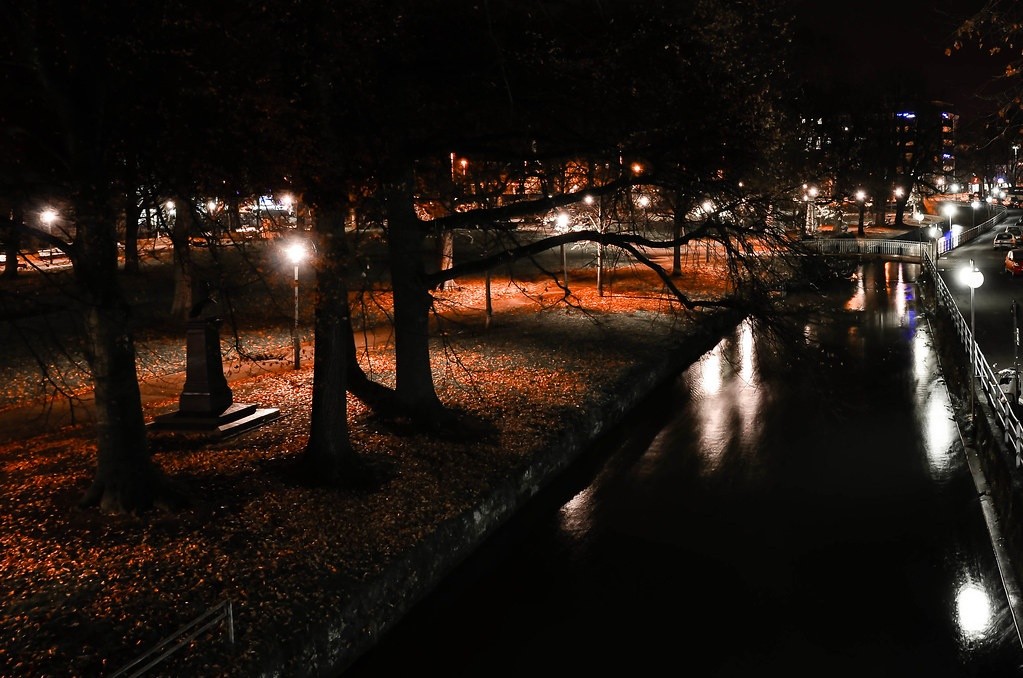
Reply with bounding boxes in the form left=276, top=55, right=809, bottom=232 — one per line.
left=915, top=213, right=925, bottom=277
left=557, top=213, right=568, bottom=298
left=945, top=204, right=955, bottom=249
left=971, top=201, right=978, bottom=230
left=41, top=210, right=53, bottom=265
left=963, top=268, right=986, bottom=433
left=284, top=240, right=309, bottom=369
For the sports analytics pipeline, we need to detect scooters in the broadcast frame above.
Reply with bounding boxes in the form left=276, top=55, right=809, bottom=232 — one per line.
left=993, top=366, right=1023, bottom=416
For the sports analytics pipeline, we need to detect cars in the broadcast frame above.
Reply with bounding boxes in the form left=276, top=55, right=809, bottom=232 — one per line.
left=1004, top=248, right=1023, bottom=277
left=993, top=232, right=1016, bottom=251
left=1004, top=215, right=1023, bottom=245
